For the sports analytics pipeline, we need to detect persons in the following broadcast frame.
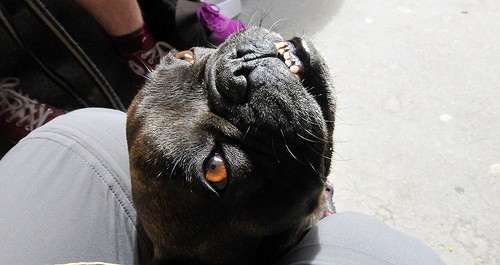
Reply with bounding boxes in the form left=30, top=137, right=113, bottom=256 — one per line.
left=0, top=0, right=249, bottom=144
left=0, top=107, right=444, bottom=265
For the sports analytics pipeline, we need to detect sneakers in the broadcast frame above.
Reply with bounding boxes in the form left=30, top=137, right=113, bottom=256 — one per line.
left=0, top=78, right=70, bottom=141
left=192, top=4, right=248, bottom=44
left=121, top=30, right=175, bottom=75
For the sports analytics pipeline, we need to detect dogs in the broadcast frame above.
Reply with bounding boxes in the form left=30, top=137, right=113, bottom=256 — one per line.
left=125, top=5, right=337, bottom=264
